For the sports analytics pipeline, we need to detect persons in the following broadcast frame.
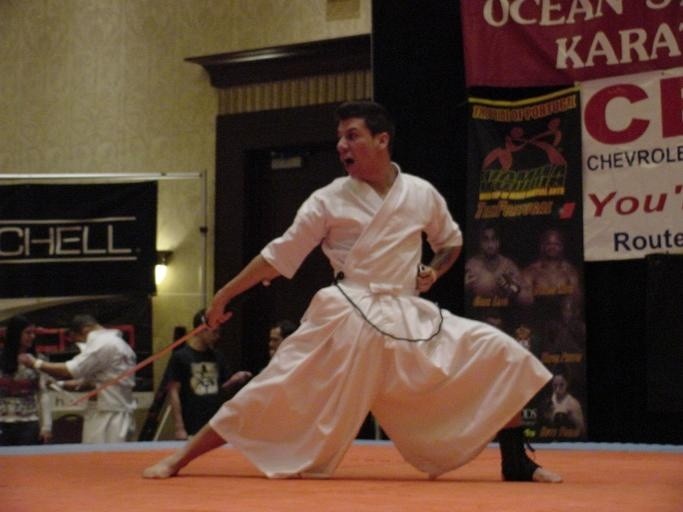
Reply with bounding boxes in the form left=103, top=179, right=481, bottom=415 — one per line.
left=141, top=98, right=567, bottom=485
left=264, top=319, right=297, bottom=359
left=165, top=308, right=254, bottom=441
left=1, top=311, right=53, bottom=444
left=14, top=312, right=136, bottom=441
left=467, top=108, right=586, bottom=441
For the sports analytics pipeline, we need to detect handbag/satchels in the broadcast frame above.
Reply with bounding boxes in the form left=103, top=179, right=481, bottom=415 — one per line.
left=32, top=358, right=43, bottom=372
